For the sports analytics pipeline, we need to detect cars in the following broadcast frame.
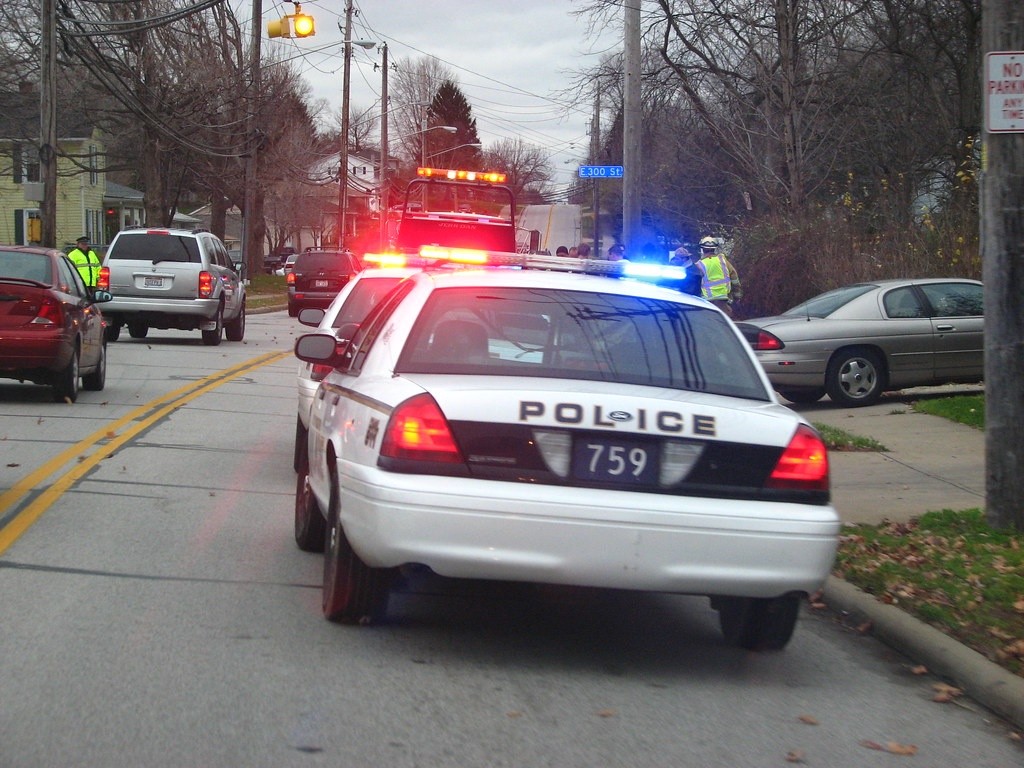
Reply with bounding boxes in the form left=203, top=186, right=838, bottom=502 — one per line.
left=225, top=249, right=241, bottom=269
left=284, top=254, right=297, bottom=275
left=295, top=243, right=842, bottom=653
left=724, top=278, right=986, bottom=407
left=268, top=246, right=295, bottom=263
left=294, top=252, right=560, bottom=473
left=1, top=244, right=114, bottom=405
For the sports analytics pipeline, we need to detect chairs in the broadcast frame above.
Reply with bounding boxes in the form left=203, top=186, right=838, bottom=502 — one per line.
left=430, top=320, right=490, bottom=358
left=898, top=294, right=918, bottom=316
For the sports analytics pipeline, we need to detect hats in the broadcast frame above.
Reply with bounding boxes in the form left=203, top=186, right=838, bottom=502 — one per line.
left=699, top=237, right=719, bottom=249
left=76, top=236, right=90, bottom=244
left=675, top=247, right=692, bottom=257
left=556, top=246, right=568, bottom=254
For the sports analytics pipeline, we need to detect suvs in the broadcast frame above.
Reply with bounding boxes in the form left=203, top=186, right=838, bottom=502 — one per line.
left=287, top=248, right=363, bottom=319
left=91, top=226, right=248, bottom=347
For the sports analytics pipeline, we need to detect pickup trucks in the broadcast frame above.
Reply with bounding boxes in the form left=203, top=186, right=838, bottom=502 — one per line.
left=263, top=255, right=282, bottom=276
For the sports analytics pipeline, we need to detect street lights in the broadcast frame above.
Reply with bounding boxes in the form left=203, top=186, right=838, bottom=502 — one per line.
left=244, top=41, right=377, bottom=283
left=421, top=142, right=482, bottom=211
left=337, top=101, right=431, bottom=247
left=379, top=126, right=457, bottom=249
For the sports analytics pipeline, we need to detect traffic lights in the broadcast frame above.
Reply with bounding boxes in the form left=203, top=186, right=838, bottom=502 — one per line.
left=266, top=19, right=290, bottom=39
left=295, top=15, right=315, bottom=37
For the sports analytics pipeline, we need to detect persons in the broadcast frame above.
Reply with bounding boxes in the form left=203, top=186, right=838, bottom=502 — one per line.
left=554, top=242, right=704, bottom=299
left=694, top=237, right=744, bottom=319
left=67, top=236, right=102, bottom=301
